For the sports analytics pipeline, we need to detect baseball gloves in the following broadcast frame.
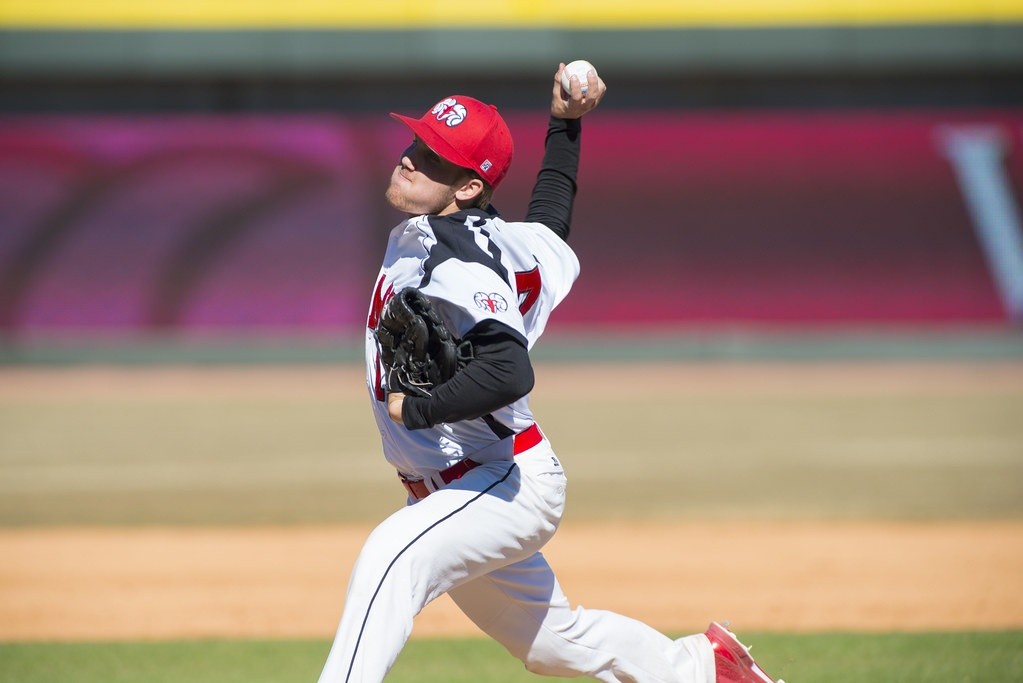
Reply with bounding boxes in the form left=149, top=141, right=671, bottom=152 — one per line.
left=372, top=286, right=460, bottom=398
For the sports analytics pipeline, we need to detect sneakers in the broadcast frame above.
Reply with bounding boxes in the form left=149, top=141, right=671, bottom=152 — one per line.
left=704, top=620, right=784, bottom=683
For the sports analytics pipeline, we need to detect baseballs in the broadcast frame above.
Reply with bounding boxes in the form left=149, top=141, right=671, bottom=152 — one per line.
left=561, top=60, right=597, bottom=97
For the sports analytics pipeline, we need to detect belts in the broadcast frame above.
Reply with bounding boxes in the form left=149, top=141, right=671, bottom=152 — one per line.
left=397, top=423, right=543, bottom=501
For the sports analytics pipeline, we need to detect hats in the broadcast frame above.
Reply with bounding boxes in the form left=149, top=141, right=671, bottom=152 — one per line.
left=391, top=94, right=515, bottom=191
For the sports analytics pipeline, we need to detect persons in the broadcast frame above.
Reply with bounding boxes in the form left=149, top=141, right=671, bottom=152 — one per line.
left=318, top=64, right=785, bottom=683
